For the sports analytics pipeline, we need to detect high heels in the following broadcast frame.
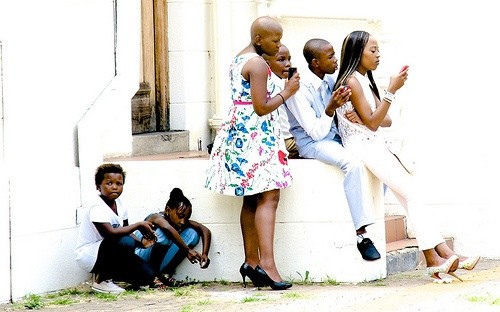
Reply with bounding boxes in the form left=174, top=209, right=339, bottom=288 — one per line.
left=446, top=255, right=481, bottom=282
left=239, top=262, right=255, bottom=288
left=426, top=254, right=460, bottom=284
left=254, top=264, right=292, bottom=291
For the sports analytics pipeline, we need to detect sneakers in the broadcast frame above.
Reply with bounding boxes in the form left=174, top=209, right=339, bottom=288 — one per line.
left=92, top=279, right=125, bottom=294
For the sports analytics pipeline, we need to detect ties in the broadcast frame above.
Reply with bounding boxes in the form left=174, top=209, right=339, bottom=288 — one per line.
left=320, top=80, right=332, bottom=109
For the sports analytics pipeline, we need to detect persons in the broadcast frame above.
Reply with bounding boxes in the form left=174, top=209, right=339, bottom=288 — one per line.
left=134, top=187, right=212, bottom=290
left=285, top=39, right=387, bottom=259
left=205, top=16, right=300, bottom=291
left=74, top=163, right=157, bottom=294
left=261, top=44, right=298, bottom=151
left=332, top=30, right=481, bottom=282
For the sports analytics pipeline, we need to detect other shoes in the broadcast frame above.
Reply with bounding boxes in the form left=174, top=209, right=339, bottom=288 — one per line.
left=149, top=277, right=166, bottom=289
left=160, top=274, right=186, bottom=288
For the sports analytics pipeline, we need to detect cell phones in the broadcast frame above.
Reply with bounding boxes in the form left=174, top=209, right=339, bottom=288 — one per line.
left=338, top=85, right=352, bottom=96
left=289, top=68, right=297, bottom=80
left=400, top=66, right=409, bottom=74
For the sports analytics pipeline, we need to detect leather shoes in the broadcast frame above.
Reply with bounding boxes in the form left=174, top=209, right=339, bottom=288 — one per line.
left=356, top=238, right=381, bottom=262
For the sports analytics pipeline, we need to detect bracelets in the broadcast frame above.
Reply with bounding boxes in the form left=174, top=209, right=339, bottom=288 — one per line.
left=383, top=98, right=392, bottom=104
left=383, top=89, right=395, bottom=101
left=277, top=93, right=284, bottom=104
